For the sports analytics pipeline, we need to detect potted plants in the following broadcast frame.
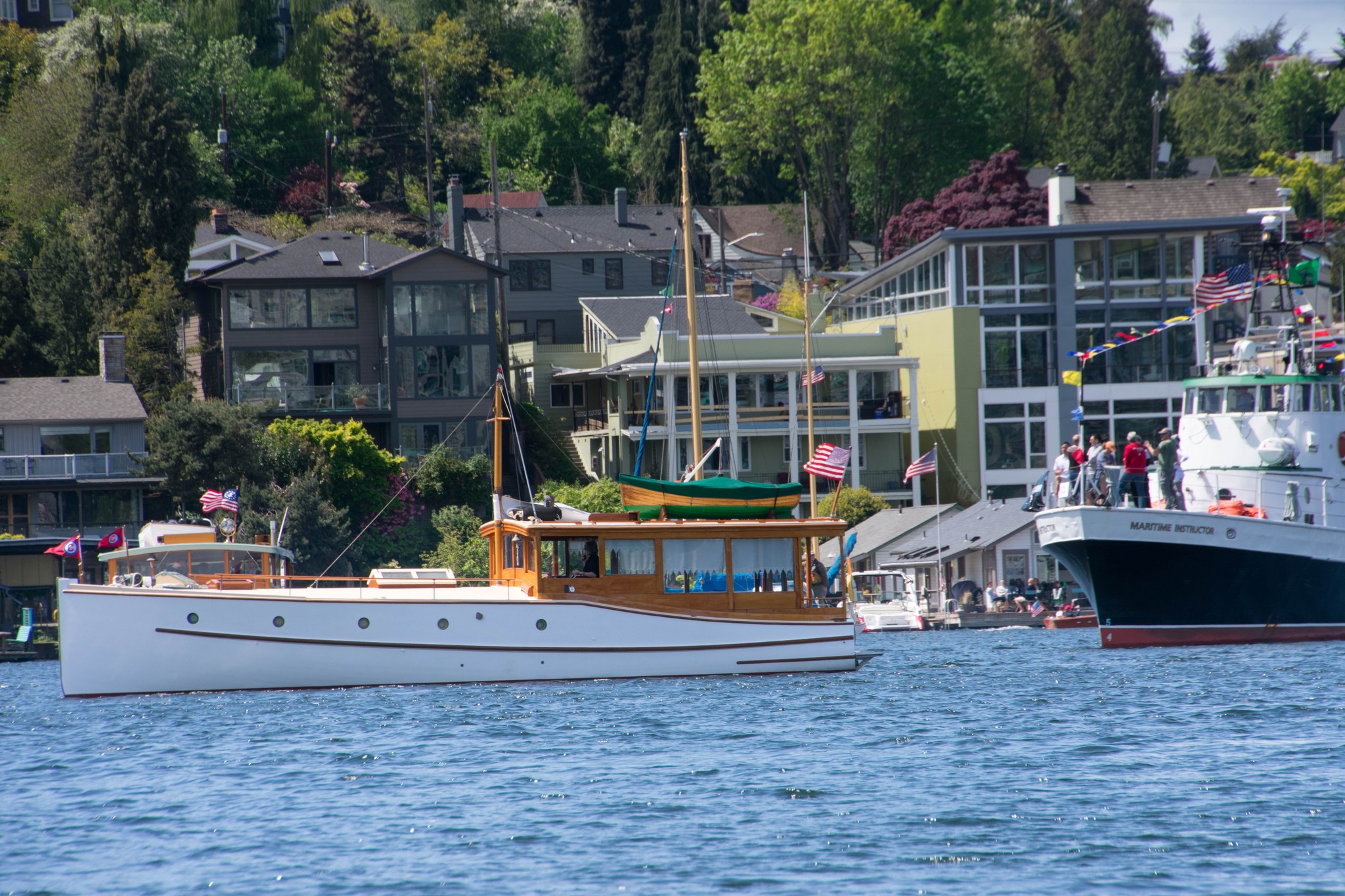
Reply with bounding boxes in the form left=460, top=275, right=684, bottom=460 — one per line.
left=957, top=589, right=986, bottom=613
left=343, top=380, right=371, bottom=408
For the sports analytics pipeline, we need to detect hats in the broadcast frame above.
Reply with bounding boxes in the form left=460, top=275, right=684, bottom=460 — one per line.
left=1105, top=441, right=1117, bottom=452
left=1137, top=435, right=1141, bottom=441
left=1158, top=427, right=1173, bottom=434
left=1127, top=431, right=1138, bottom=442
left=1170, top=434, right=1180, bottom=446
left=811, top=550, right=816, bottom=555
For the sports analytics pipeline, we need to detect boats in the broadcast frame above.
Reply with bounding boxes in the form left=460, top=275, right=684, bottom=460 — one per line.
left=1021, top=186, right=1345, bottom=650
left=842, top=569, right=926, bottom=632
left=56, top=125, right=887, bottom=704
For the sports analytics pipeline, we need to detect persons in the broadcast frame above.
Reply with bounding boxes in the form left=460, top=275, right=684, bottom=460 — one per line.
left=245, top=558, right=262, bottom=575
left=1086, top=433, right=1117, bottom=505
left=1066, top=434, right=1088, bottom=505
left=985, top=578, right=1064, bottom=613
left=1229, top=386, right=1290, bottom=412
left=1118, top=431, right=1151, bottom=508
left=166, top=558, right=189, bottom=578
left=572, top=540, right=599, bottom=578
left=803, top=550, right=828, bottom=608
left=1053, top=441, right=1072, bottom=507
left=1056, top=604, right=1080, bottom=618
left=1143, top=427, right=1186, bottom=511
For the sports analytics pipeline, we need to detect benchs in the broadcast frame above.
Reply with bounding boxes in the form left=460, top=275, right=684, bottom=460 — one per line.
left=3, top=625, right=33, bottom=652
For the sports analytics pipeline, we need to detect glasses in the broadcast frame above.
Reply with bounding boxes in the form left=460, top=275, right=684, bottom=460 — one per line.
left=1162, top=433, right=1165, bottom=435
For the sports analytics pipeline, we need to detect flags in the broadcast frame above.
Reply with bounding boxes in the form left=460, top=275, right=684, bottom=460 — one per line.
left=1190, top=260, right=1252, bottom=305
left=1240, top=261, right=1289, bottom=296
left=660, top=302, right=673, bottom=315
left=903, top=446, right=936, bottom=484
left=1062, top=370, right=1084, bottom=421
left=1290, top=304, right=1345, bottom=363
left=659, top=285, right=672, bottom=299
left=43, top=533, right=82, bottom=559
left=802, top=442, right=851, bottom=481
left=1281, top=257, right=1320, bottom=286
left=199, top=488, right=238, bottom=514
left=801, top=365, right=825, bottom=386
left=1030, top=601, right=1045, bottom=618
left=1065, top=295, right=1234, bottom=362
left=98, top=526, right=125, bottom=548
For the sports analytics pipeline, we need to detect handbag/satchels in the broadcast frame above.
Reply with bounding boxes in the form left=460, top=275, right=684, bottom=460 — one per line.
left=811, top=572, right=823, bottom=583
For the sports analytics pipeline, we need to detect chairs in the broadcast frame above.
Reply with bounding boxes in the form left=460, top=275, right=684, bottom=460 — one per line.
left=313, top=389, right=332, bottom=411
